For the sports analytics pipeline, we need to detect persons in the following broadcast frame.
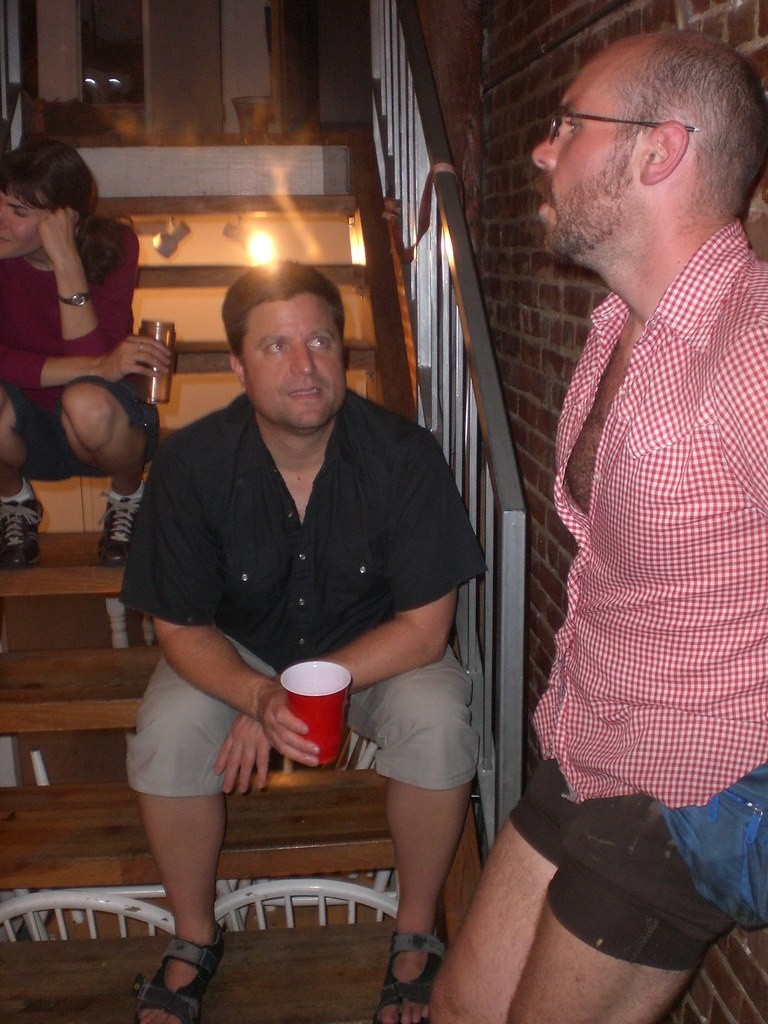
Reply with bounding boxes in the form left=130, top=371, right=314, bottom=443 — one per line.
left=0, top=138, right=172, bottom=566
left=117, top=258, right=487, bottom=1024
left=430, top=31, right=768, bottom=1023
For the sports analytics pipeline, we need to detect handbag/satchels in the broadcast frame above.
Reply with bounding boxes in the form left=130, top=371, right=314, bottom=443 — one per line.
left=659, top=763, right=768, bottom=930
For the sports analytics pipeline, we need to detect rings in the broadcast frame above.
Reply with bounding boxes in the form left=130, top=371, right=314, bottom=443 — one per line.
left=140, top=342, right=143, bottom=351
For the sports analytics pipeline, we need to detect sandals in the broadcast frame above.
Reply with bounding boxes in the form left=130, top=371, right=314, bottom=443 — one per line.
left=132, top=920, right=225, bottom=1024
left=371, top=926, right=446, bottom=1024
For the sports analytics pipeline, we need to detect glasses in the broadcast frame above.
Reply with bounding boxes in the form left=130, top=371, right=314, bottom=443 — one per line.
left=542, top=102, right=702, bottom=147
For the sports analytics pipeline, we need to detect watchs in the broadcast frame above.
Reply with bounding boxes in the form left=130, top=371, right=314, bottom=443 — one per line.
left=58, top=291, right=91, bottom=307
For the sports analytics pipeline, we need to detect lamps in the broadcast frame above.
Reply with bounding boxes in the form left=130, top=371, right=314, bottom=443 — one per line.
left=222, top=215, right=277, bottom=265
left=153, top=215, right=191, bottom=257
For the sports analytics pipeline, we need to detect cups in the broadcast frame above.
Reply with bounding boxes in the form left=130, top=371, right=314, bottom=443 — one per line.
left=280, top=660, right=352, bottom=764
left=133, top=320, right=176, bottom=404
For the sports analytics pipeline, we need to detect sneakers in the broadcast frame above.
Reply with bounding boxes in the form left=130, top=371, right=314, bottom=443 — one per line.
left=0, top=475, right=43, bottom=568
left=98, top=480, right=146, bottom=567
